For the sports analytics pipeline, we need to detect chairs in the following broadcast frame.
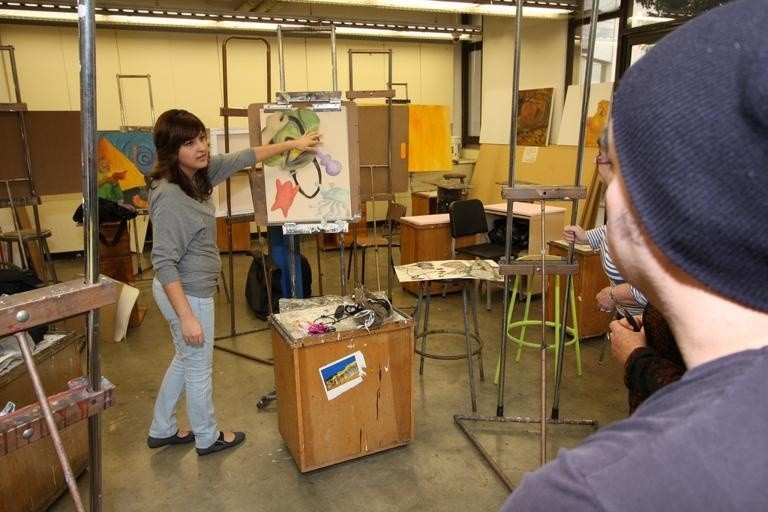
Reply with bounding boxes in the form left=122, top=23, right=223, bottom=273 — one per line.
left=449, top=197, right=526, bottom=312
left=346, top=199, right=409, bottom=286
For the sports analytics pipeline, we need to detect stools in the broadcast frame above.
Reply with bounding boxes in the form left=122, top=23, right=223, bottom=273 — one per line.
left=0, top=228, right=60, bottom=288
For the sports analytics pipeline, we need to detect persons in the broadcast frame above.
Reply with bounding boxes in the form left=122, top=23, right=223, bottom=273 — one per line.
left=609, top=302, right=687, bottom=417
left=147, top=109, right=325, bottom=455
left=501, top=1, right=766, bottom=511
left=565, top=129, right=648, bottom=321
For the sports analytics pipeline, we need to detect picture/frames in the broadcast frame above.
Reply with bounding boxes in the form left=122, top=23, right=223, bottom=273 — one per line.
left=516, top=85, right=554, bottom=148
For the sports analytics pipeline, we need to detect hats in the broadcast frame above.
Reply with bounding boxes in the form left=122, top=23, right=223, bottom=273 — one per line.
left=611, top=1, right=767, bottom=315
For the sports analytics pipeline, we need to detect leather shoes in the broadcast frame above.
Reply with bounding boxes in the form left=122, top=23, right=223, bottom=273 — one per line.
left=147, top=430, right=194, bottom=448
left=196, top=431, right=245, bottom=455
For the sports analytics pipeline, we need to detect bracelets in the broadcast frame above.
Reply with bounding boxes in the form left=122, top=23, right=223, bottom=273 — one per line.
left=609, top=283, right=616, bottom=300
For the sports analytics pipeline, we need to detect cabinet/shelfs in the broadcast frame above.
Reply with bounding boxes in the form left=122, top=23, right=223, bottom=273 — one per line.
left=399, top=214, right=472, bottom=298
left=410, top=189, right=469, bottom=215
left=266, top=303, right=416, bottom=474
left=479, top=201, right=565, bottom=298
left=547, top=239, right=616, bottom=341
